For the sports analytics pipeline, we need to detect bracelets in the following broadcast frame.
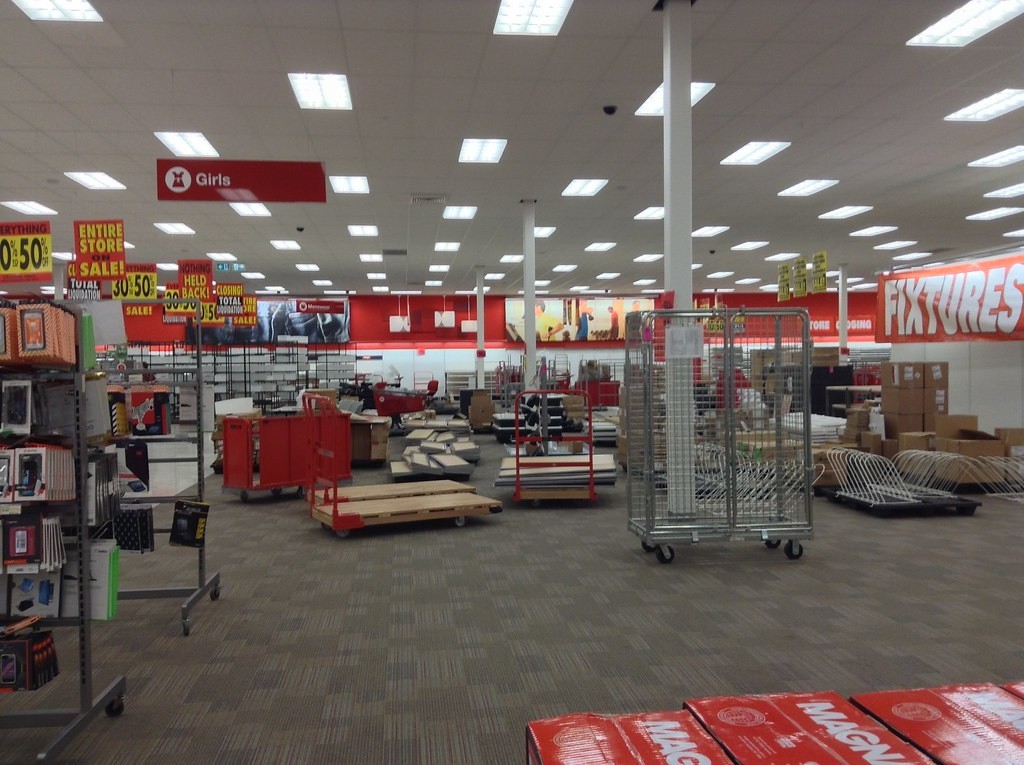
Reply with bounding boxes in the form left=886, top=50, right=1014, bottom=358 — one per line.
left=549, top=332, right=552, bottom=335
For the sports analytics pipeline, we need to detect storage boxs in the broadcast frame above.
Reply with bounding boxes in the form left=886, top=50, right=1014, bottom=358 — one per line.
left=847, top=681, right=1024, bottom=765
left=924, top=388, right=949, bottom=415
left=797, top=449, right=848, bottom=469
left=898, top=432, right=936, bottom=476
left=683, top=690, right=936, bottom=765
left=881, top=361, right=924, bottom=389
left=305, top=388, right=337, bottom=411
left=995, top=428, right=1024, bottom=482
left=882, top=411, right=923, bottom=439
left=924, top=414, right=949, bottom=432
left=880, top=386, right=924, bottom=415
left=615, top=346, right=840, bottom=470
left=216, top=408, right=262, bottom=432
left=923, top=361, right=949, bottom=389
left=861, top=432, right=882, bottom=455
left=800, top=470, right=846, bottom=487
left=935, top=413, right=1005, bottom=483
left=526, top=708, right=734, bottom=765
left=881, top=439, right=898, bottom=459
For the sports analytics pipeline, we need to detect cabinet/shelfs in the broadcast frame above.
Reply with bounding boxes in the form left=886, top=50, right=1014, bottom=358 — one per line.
left=443, top=369, right=621, bottom=406
left=351, top=415, right=390, bottom=470
left=128, top=343, right=355, bottom=417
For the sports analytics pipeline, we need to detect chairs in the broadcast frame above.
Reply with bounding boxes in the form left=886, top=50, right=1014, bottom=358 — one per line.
left=427, top=381, right=438, bottom=400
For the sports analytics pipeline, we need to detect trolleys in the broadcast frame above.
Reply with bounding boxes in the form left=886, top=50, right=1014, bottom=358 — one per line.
left=302, top=393, right=502, bottom=536
left=222, top=393, right=352, bottom=501
left=625, top=307, right=815, bottom=564
left=512, top=389, right=598, bottom=503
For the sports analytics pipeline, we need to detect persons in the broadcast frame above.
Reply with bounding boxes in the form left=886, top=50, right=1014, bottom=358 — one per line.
left=607, top=306, right=619, bottom=340
left=625, top=301, right=642, bottom=338
left=535, top=300, right=564, bottom=342
left=576, top=306, right=593, bottom=340
left=141, top=362, right=156, bottom=381
